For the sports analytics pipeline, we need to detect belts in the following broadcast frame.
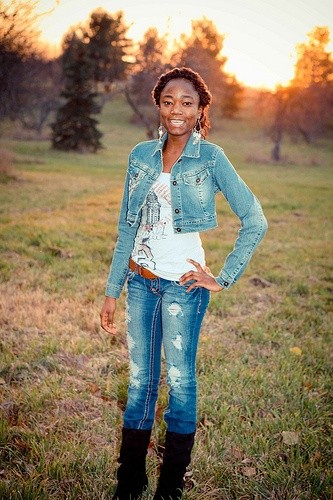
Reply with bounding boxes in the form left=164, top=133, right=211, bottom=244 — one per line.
left=129, top=258, right=158, bottom=279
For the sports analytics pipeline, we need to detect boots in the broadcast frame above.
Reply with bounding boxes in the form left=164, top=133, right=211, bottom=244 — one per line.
left=111, top=427, right=196, bottom=500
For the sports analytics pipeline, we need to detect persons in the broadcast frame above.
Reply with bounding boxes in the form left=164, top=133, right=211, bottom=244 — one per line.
left=99, top=68, right=268, bottom=500
